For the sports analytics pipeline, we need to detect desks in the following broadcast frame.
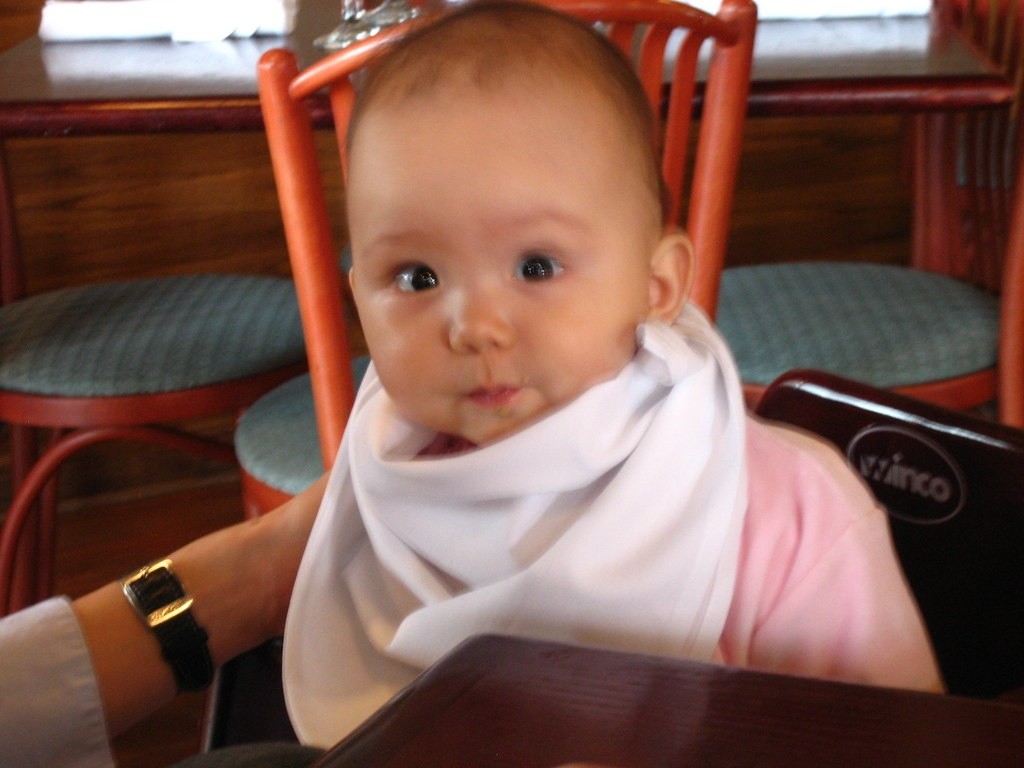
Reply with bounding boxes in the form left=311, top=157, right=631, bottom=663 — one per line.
left=310, top=626, right=1024, bottom=768
left=2, top=15, right=1015, bottom=137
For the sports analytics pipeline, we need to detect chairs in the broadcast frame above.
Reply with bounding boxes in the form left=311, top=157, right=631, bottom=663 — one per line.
left=0, top=0, right=1024, bottom=768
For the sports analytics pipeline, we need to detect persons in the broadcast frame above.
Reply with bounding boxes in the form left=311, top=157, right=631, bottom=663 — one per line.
left=281, top=0, right=944, bottom=751
left=0, top=471, right=332, bottom=768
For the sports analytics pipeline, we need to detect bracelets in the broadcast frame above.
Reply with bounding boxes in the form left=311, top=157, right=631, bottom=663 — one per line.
left=120, top=558, right=215, bottom=692
